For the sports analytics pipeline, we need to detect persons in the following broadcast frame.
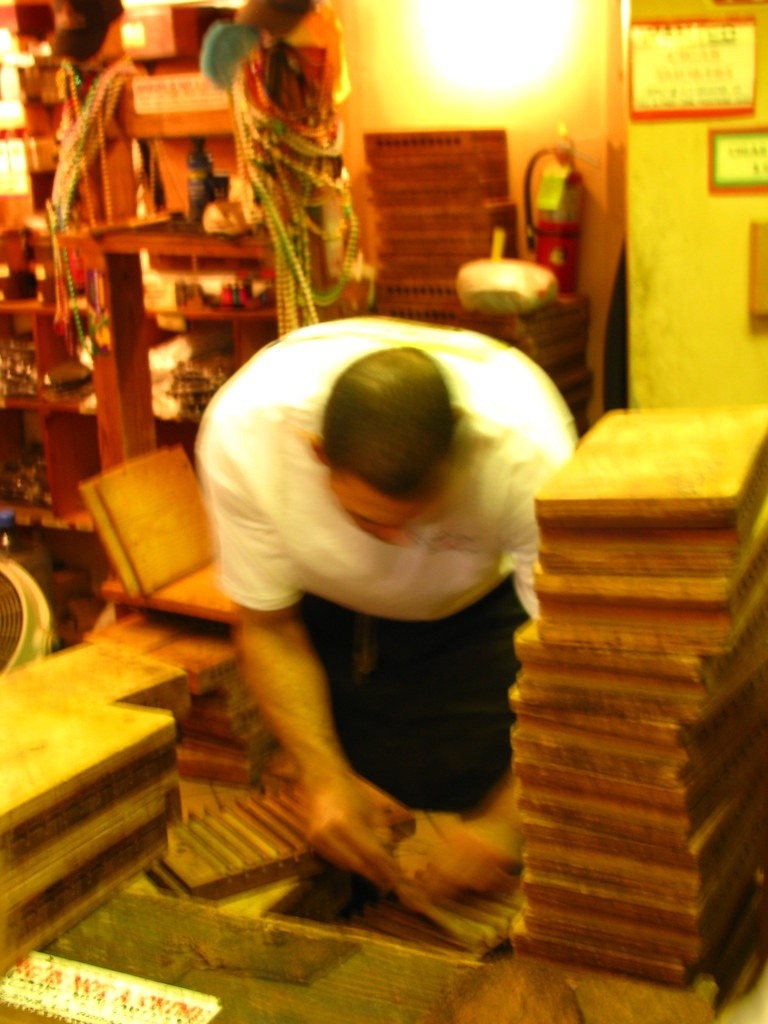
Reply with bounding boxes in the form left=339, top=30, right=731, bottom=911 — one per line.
left=193, top=316, right=579, bottom=899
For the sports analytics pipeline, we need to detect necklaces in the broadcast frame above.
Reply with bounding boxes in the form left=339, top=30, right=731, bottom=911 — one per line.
left=33, top=58, right=136, bottom=356
left=231, top=42, right=357, bottom=344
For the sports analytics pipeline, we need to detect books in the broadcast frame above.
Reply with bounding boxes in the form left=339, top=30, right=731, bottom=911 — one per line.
left=508, top=403, right=768, bottom=988
left=0, top=612, right=268, bottom=978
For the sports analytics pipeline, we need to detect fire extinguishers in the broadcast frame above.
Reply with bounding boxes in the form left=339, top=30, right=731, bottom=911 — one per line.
left=520, top=124, right=587, bottom=294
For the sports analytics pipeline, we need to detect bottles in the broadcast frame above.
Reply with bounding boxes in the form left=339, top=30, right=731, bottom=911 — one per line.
left=1, top=509, right=58, bottom=620
left=186, top=136, right=212, bottom=221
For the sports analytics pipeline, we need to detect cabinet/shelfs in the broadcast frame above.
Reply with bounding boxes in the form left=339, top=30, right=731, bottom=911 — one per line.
left=0, top=0, right=338, bottom=531
